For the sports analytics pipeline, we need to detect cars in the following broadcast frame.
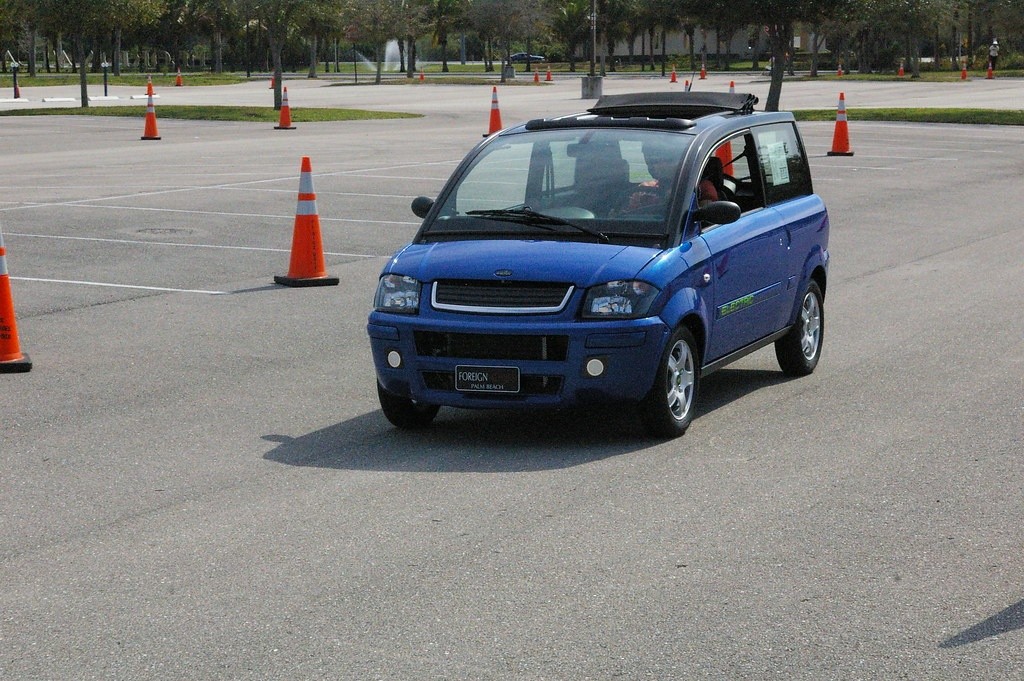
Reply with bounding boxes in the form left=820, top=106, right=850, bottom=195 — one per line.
left=366, top=92, right=830, bottom=440
left=510, top=52, right=546, bottom=64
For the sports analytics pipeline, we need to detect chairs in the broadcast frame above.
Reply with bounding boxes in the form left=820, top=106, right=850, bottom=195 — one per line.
left=706, top=157, right=729, bottom=201
left=576, top=157, right=632, bottom=211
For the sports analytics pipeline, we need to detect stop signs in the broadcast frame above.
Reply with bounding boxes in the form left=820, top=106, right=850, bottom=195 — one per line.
left=346, top=25, right=360, bottom=43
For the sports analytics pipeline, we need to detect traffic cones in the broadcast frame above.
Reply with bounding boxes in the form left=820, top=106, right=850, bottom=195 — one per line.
left=139, top=86, right=162, bottom=141
left=143, top=75, right=157, bottom=95
left=532, top=66, right=539, bottom=83
left=826, top=92, right=856, bottom=157
left=985, top=61, right=995, bottom=79
left=268, top=67, right=275, bottom=89
left=482, top=86, right=503, bottom=138
left=684, top=79, right=690, bottom=92
left=698, top=63, right=708, bottom=79
left=0, top=231, right=34, bottom=373
left=714, top=141, right=735, bottom=178
left=669, top=64, right=679, bottom=83
left=960, top=62, right=967, bottom=80
left=419, top=67, right=426, bottom=81
left=273, top=87, right=297, bottom=130
left=273, top=155, right=340, bottom=288
left=897, top=62, right=905, bottom=77
left=15, top=81, right=21, bottom=98
left=728, top=81, right=736, bottom=93
left=174, top=67, right=184, bottom=87
left=836, top=63, right=843, bottom=76
left=543, top=65, right=555, bottom=81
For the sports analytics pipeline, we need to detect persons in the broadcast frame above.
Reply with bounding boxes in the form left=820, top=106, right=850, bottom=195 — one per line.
left=608, top=141, right=718, bottom=229
left=988, top=41, right=999, bottom=71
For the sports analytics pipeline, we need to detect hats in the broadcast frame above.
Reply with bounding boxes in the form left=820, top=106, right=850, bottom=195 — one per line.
left=993, top=41, right=998, bottom=44
